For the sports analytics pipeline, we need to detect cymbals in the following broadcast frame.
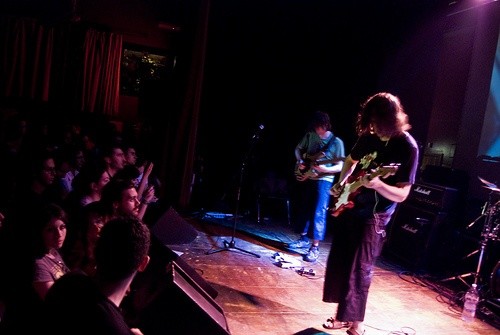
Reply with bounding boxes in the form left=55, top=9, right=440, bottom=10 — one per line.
left=482, top=185, right=500, bottom=192
left=478, top=176, right=497, bottom=188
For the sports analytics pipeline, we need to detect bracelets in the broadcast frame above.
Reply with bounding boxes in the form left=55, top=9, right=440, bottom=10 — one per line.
left=141, top=199, right=152, bottom=205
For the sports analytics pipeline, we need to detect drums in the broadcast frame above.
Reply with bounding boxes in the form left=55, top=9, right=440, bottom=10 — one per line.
left=489, top=261, right=500, bottom=301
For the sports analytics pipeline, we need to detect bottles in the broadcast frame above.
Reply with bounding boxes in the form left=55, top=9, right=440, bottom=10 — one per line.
left=462, top=283, right=479, bottom=322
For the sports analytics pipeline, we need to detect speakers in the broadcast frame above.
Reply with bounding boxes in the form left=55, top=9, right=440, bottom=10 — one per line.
left=127, top=238, right=231, bottom=335
left=387, top=203, right=458, bottom=275
left=147, top=205, right=199, bottom=245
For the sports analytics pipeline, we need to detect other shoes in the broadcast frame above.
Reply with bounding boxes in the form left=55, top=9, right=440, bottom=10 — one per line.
left=323, top=316, right=354, bottom=329
left=306, top=245, right=320, bottom=262
left=346, top=326, right=366, bottom=335
left=288, top=237, right=310, bottom=247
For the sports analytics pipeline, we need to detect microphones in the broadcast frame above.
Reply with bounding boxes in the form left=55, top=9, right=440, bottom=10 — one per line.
left=251, top=125, right=264, bottom=139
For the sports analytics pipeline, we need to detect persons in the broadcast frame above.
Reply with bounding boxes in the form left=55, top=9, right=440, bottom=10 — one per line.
left=99, top=179, right=155, bottom=221
left=322, top=91, right=419, bottom=335
left=70, top=201, right=110, bottom=277
left=283, top=111, right=345, bottom=262
left=62, top=168, right=112, bottom=208
left=39, top=217, right=151, bottom=335
left=26, top=203, right=72, bottom=299
left=122, top=145, right=147, bottom=179
left=0, top=110, right=139, bottom=165
left=62, top=147, right=86, bottom=193
left=99, top=143, right=154, bottom=201
left=19, top=142, right=64, bottom=224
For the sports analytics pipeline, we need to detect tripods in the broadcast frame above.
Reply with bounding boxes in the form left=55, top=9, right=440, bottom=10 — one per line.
left=206, top=137, right=260, bottom=258
left=438, top=190, right=495, bottom=303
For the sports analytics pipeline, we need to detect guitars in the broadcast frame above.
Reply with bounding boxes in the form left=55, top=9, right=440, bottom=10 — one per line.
left=331, top=163, right=401, bottom=217
left=294, top=151, right=347, bottom=181
